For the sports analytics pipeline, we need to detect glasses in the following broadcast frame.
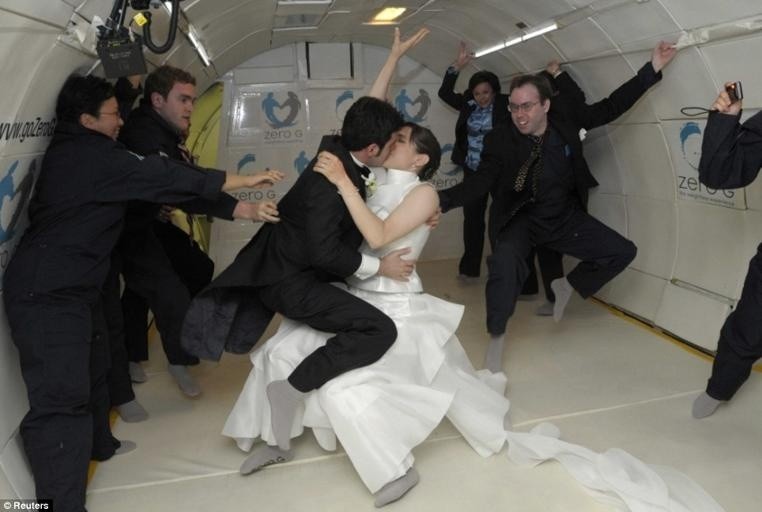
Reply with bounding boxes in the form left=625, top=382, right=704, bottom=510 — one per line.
left=508, top=101, right=542, bottom=112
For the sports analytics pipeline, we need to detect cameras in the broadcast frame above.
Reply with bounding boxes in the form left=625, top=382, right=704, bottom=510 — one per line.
left=725, top=81, right=744, bottom=102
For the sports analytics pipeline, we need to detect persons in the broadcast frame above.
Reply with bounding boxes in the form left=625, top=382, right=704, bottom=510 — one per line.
left=691, top=82, right=762, bottom=420
